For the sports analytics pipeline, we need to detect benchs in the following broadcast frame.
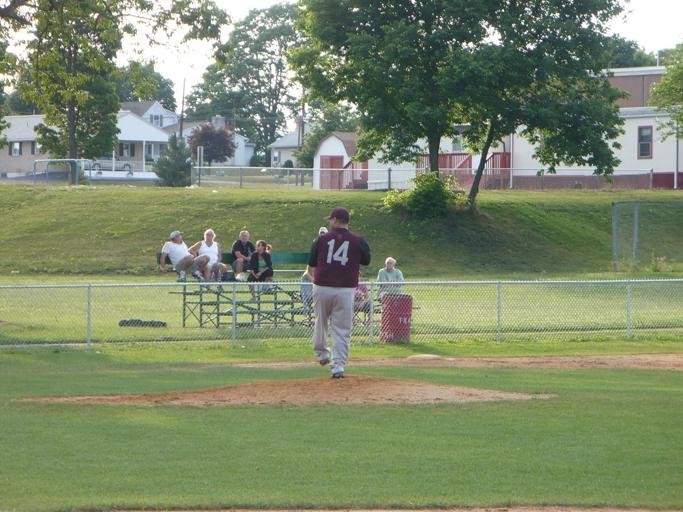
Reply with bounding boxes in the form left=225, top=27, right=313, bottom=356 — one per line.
left=157, top=249, right=420, bottom=330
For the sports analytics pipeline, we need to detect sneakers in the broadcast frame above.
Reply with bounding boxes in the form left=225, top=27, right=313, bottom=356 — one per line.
left=319, top=348, right=343, bottom=378
left=179, top=273, right=203, bottom=281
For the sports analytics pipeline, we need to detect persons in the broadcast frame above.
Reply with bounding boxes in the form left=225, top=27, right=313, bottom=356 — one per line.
left=317, top=226, right=328, bottom=236
left=304, top=206, right=370, bottom=377
left=298, top=264, right=314, bottom=303
left=375, top=256, right=406, bottom=306
left=230, top=230, right=256, bottom=282
left=186, top=227, right=226, bottom=282
left=351, top=270, right=374, bottom=325
left=158, top=230, right=211, bottom=282
left=245, top=240, right=272, bottom=303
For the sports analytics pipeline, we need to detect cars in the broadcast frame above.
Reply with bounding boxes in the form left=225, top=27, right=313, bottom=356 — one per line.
left=272, top=175, right=287, bottom=184
left=260, top=169, right=272, bottom=176
left=82, top=152, right=135, bottom=172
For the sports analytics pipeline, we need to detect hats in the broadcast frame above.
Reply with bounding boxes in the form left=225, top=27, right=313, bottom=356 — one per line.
left=170, top=231, right=183, bottom=239
left=319, top=227, right=328, bottom=234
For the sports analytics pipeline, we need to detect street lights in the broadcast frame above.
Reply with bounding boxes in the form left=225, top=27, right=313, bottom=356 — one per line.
left=294, top=115, right=303, bottom=185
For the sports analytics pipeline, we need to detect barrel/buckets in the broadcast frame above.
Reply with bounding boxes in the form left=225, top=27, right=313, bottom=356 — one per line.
left=382, top=296, right=412, bottom=343
